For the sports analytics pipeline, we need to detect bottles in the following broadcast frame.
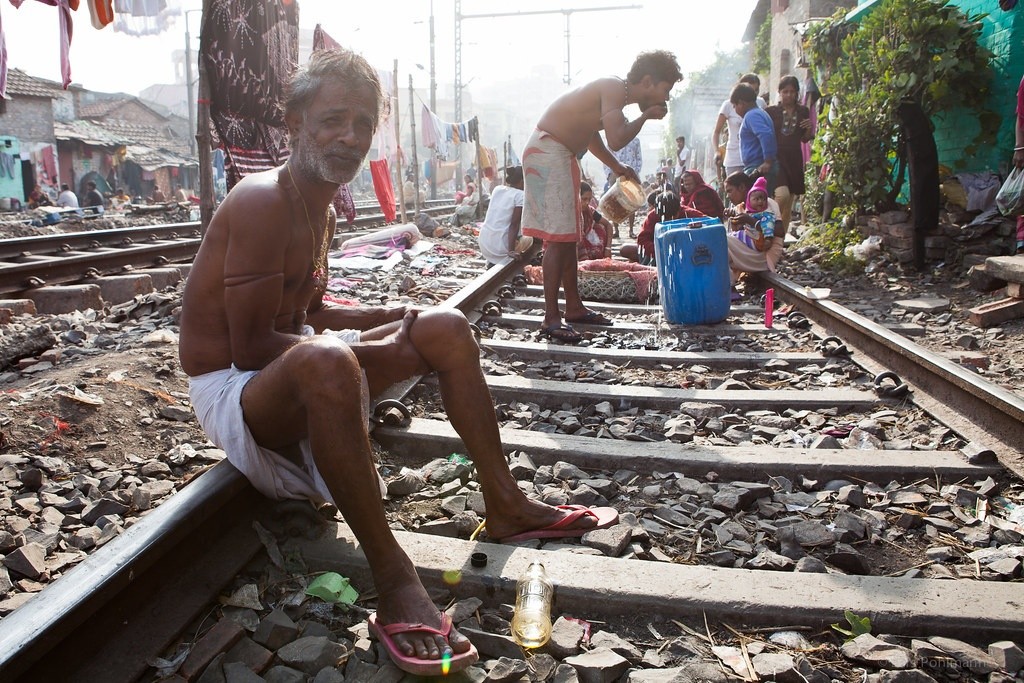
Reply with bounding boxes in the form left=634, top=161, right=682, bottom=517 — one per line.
left=511, top=563, right=552, bottom=649
left=764, top=288, right=774, bottom=328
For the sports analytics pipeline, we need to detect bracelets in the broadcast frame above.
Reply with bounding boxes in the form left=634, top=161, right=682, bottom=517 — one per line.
left=605, top=247, right=611, bottom=250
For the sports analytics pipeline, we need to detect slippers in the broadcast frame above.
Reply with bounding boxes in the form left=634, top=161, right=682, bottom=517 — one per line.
left=540, top=324, right=582, bottom=341
left=500, top=505, right=620, bottom=540
left=370, top=611, right=480, bottom=675
left=566, top=313, right=613, bottom=325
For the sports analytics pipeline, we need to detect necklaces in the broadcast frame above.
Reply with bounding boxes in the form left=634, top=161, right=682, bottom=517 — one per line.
left=625, top=81, right=628, bottom=104
left=285, top=161, right=323, bottom=278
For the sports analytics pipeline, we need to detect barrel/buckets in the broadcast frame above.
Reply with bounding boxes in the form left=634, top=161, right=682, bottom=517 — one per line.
left=654, top=216, right=721, bottom=253
left=657, top=222, right=730, bottom=324
left=600, top=176, right=646, bottom=224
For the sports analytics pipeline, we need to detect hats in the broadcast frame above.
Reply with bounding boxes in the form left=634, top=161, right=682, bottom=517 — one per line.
left=746, top=177, right=768, bottom=212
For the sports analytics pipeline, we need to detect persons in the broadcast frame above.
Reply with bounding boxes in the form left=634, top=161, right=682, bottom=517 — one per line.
left=178, top=48, right=598, bottom=676
left=621, top=170, right=785, bottom=283
left=466, top=162, right=476, bottom=177
left=113, top=188, right=129, bottom=204
left=521, top=49, right=683, bottom=343
left=152, top=184, right=166, bottom=202
left=455, top=175, right=476, bottom=205
left=175, top=184, right=187, bottom=201
left=31, top=184, right=41, bottom=201
left=57, top=183, right=78, bottom=207
left=405, top=173, right=428, bottom=209
left=83, top=181, right=103, bottom=206
left=658, top=136, right=690, bottom=188
left=578, top=181, right=613, bottom=260
left=478, top=165, right=533, bottom=269
left=603, top=137, right=642, bottom=238
left=712, top=73, right=812, bottom=233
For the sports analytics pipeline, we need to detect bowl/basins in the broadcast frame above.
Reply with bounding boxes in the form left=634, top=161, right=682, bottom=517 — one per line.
left=806, top=288, right=831, bottom=300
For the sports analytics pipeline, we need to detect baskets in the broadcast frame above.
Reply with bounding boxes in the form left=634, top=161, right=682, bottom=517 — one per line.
left=576, top=260, right=658, bottom=300
